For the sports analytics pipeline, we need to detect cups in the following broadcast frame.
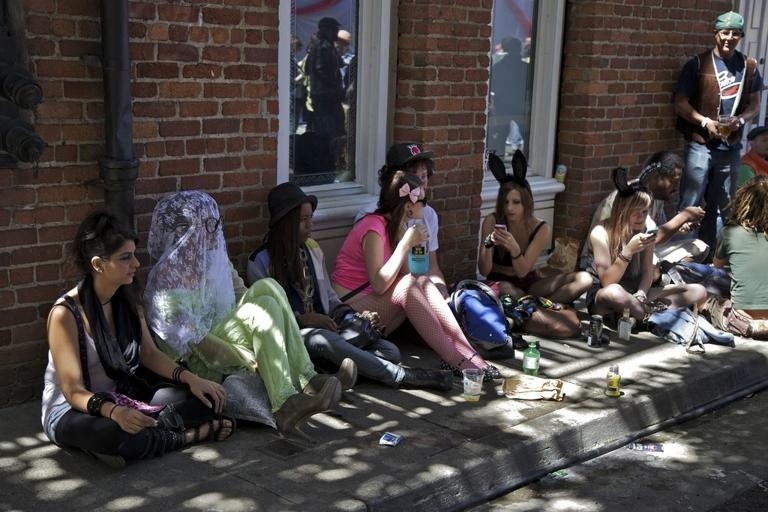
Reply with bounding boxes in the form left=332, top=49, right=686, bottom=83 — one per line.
left=462, top=367, right=484, bottom=402
left=717, top=114, right=731, bottom=137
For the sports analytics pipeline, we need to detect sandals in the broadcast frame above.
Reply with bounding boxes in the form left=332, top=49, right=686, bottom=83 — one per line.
left=440, top=356, right=493, bottom=383
left=469, top=352, right=503, bottom=380
left=90, top=450, right=126, bottom=470
left=191, top=414, right=237, bottom=443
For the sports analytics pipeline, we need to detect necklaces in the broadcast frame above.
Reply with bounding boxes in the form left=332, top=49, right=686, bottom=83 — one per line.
left=99, top=298, right=111, bottom=307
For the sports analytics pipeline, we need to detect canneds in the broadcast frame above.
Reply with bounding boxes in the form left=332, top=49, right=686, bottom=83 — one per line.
left=587, top=315, right=604, bottom=348
left=555, top=164, right=567, bottom=183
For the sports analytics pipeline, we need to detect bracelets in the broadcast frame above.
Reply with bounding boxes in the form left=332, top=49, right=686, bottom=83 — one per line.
left=510, top=250, right=525, bottom=261
left=170, top=363, right=187, bottom=384
left=86, top=391, right=122, bottom=419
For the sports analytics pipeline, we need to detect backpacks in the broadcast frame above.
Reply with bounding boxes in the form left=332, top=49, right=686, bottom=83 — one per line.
left=446, top=277, right=516, bottom=362
left=645, top=302, right=736, bottom=355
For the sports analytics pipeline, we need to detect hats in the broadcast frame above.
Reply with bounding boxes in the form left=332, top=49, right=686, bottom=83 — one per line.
left=712, top=10, right=745, bottom=32
left=385, top=141, right=434, bottom=171
left=267, top=181, right=318, bottom=229
left=746, top=126, right=768, bottom=142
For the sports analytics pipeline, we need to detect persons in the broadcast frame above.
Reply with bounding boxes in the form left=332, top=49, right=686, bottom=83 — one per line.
left=583, top=122, right=768, bottom=346
left=486, top=30, right=540, bottom=174
left=41, top=210, right=236, bottom=467
left=477, top=149, right=594, bottom=336
left=332, top=167, right=502, bottom=385
left=144, top=190, right=355, bottom=449
left=247, top=181, right=450, bottom=406
left=380, top=141, right=476, bottom=347
left=670, top=11, right=764, bottom=241
left=287, top=15, right=363, bottom=175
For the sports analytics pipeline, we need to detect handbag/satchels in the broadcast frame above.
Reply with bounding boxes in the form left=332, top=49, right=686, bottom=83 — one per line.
left=326, top=302, right=381, bottom=351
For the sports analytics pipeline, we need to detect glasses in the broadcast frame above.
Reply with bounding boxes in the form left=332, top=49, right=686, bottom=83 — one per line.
left=204, top=214, right=227, bottom=236
left=415, top=196, right=428, bottom=207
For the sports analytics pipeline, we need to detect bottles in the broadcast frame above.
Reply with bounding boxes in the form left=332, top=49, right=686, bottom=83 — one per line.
left=605, top=362, right=621, bottom=397
left=553, top=164, right=567, bottom=183
left=522, top=337, right=541, bottom=375
left=616, top=307, right=632, bottom=341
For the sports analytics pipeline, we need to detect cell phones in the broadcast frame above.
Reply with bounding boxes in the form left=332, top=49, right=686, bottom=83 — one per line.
left=647, top=229, right=659, bottom=236
left=494, top=223, right=508, bottom=246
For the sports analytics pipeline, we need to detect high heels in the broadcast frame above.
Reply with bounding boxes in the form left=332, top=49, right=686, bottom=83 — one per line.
left=399, top=367, right=454, bottom=393
left=301, top=357, right=358, bottom=405
left=274, top=376, right=342, bottom=450
